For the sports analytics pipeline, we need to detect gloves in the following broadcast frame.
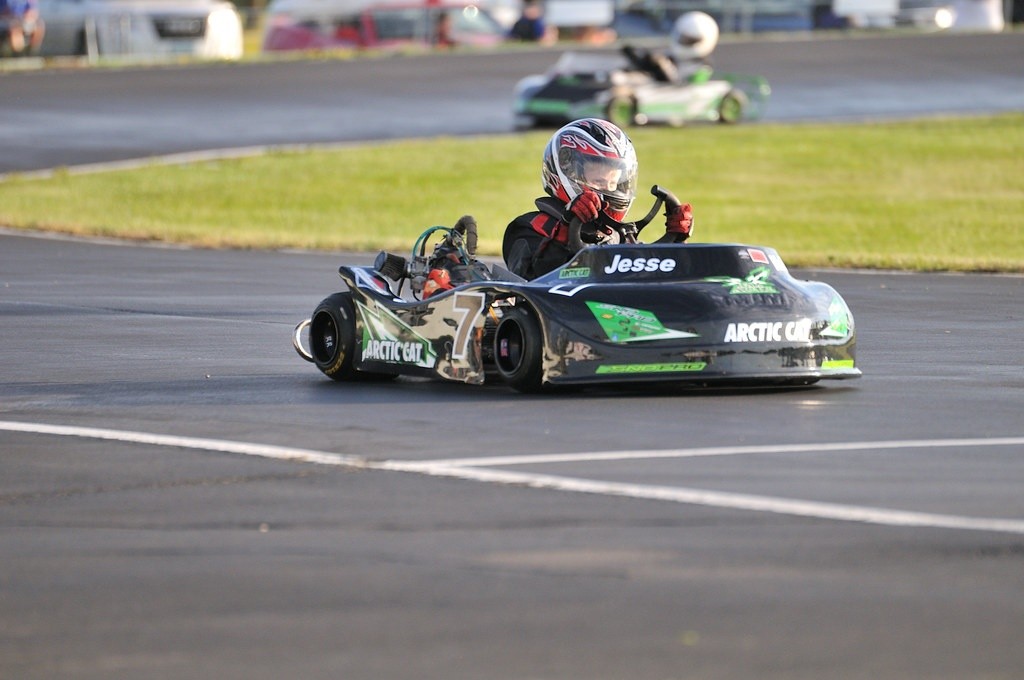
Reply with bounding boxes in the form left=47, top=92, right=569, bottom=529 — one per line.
left=663, top=203, right=694, bottom=233
left=561, top=190, right=610, bottom=224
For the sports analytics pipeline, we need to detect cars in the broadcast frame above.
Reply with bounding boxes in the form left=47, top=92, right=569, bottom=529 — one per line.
left=261, top=1, right=513, bottom=49
left=621, top=0, right=846, bottom=37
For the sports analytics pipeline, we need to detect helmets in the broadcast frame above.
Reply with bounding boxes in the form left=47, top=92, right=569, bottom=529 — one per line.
left=669, top=11, right=720, bottom=60
left=542, top=118, right=638, bottom=223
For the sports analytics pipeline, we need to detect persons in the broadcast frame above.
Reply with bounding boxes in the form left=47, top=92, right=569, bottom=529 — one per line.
left=503, top=117, right=693, bottom=282
left=624, top=10, right=719, bottom=83
left=511, top=0, right=558, bottom=43
left=435, top=12, right=457, bottom=49
left=0, top=0, right=45, bottom=59
